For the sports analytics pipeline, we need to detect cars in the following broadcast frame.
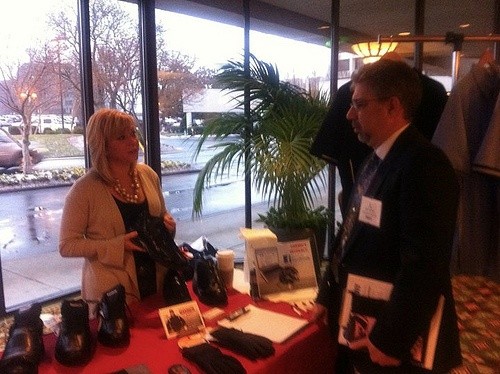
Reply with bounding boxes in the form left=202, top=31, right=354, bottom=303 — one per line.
left=0, top=127, right=43, bottom=167
left=0, top=113, right=79, bottom=133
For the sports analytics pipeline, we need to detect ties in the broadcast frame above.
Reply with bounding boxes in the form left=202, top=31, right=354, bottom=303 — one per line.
left=330, top=149, right=381, bottom=286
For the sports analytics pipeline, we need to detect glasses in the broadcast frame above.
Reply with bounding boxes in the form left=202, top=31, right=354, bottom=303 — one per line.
left=350, top=95, right=385, bottom=110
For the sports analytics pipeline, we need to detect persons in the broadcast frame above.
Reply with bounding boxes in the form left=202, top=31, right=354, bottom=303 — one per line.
left=167, top=309, right=187, bottom=333
left=327, top=59, right=462, bottom=374
left=58, top=109, right=176, bottom=318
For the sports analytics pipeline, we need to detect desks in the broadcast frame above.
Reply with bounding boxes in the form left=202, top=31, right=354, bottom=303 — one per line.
left=0, top=262, right=328, bottom=374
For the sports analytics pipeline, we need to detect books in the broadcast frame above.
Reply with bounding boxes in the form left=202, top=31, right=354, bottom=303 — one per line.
left=338, top=273, right=445, bottom=369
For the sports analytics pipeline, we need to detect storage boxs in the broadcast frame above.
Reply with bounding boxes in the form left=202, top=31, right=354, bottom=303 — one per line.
left=241, top=228, right=279, bottom=292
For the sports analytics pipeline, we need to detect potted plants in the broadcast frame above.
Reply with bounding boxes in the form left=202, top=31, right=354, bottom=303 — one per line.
left=186, top=52, right=329, bottom=262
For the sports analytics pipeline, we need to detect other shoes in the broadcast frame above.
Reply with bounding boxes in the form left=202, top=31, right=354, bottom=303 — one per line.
left=92, top=283, right=141, bottom=346
left=54, top=298, right=94, bottom=368
left=130, top=212, right=190, bottom=267
left=162, top=269, right=192, bottom=305
left=192, top=254, right=228, bottom=306
left=0, top=303, right=47, bottom=374
left=178, top=239, right=218, bottom=282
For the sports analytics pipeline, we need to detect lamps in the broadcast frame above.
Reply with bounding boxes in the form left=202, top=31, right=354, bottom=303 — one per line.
left=350, top=38, right=399, bottom=64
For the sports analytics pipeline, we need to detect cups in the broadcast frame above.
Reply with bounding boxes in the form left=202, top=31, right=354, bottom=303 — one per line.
left=215, top=249, right=234, bottom=291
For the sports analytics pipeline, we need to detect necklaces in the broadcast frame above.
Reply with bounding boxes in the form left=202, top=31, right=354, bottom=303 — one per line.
left=114, top=171, right=140, bottom=204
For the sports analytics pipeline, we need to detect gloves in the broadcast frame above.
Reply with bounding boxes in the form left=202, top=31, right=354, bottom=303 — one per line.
left=211, top=327, right=274, bottom=359
left=182, top=341, right=248, bottom=374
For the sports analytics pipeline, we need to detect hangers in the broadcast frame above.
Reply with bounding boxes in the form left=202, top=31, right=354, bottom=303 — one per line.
left=476, top=32, right=500, bottom=68
left=378, top=35, right=402, bottom=63
